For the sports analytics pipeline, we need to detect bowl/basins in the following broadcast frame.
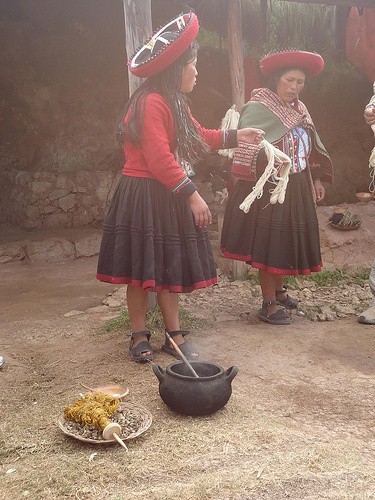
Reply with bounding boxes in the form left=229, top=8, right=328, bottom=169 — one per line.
left=89, top=386, right=129, bottom=402
left=356, top=192, right=371, bottom=203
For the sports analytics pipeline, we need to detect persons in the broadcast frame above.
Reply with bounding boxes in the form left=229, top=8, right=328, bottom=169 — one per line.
left=358, top=81, right=375, bottom=325
left=96, top=10, right=266, bottom=361
left=219, top=48, right=332, bottom=324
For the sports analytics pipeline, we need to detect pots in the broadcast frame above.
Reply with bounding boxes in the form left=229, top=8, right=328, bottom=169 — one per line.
left=152, top=360, right=239, bottom=416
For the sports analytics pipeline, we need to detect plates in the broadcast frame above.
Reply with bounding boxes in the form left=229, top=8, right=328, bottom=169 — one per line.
left=57, top=402, right=152, bottom=444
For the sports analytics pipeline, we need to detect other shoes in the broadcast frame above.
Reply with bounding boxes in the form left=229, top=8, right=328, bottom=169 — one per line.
left=358, top=306, right=375, bottom=323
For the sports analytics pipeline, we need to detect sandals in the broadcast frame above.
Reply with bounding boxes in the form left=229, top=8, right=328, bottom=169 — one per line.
left=257, top=301, right=293, bottom=325
left=129, top=331, right=157, bottom=362
left=276, top=290, right=298, bottom=309
left=164, top=330, right=199, bottom=360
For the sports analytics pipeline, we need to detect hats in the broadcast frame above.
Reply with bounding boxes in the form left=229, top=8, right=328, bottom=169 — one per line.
left=126, top=10, right=200, bottom=78
left=257, top=48, right=325, bottom=77
